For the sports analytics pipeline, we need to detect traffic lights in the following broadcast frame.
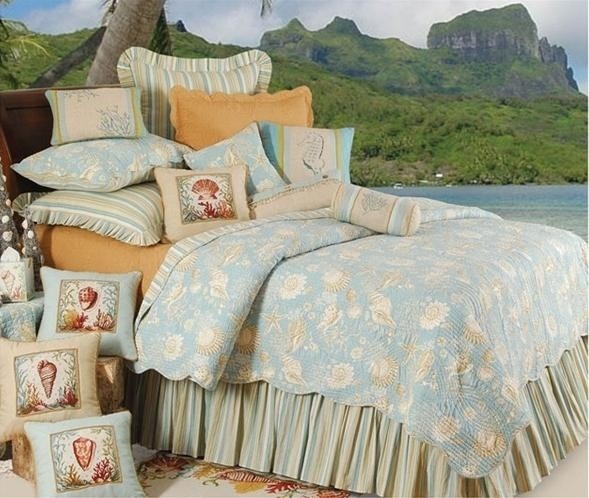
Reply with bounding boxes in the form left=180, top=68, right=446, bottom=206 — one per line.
left=34, top=265, right=143, bottom=361
left=170, top=85, right=314, bottom=151
left=181, top=121, right=288, bottom=198
left=260, top=121, right=355, bottom=187
left=10, top=182, right=163, bottom=247
left=116, top=46, right=272, bottom=139
left=10, top=134, right=196, bottom=192
left=155, top=165, right=251, bottom=243
left=22, top=408, right=147, bottom=497
left=0, top=329, right=102, bottom=444
left=249, top=169, right=342, bottom=220
left=46, top=86, right=150, bottom=146
left=330, top=182, right=422, bottom=238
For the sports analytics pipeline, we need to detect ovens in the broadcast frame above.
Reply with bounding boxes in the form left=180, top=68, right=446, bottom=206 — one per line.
left=0, top=86, right=588, bottom=497
